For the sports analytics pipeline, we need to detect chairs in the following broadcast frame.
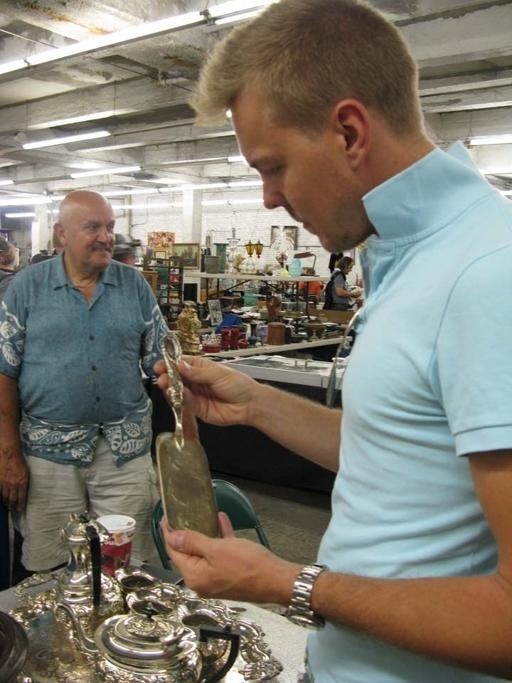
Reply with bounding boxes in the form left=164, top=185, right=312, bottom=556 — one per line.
left=152, top=479, right=272, bottom=601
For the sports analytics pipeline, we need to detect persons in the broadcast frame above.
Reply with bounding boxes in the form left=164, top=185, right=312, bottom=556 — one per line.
left=0, top=190, right=200, bottom=575
left=168, top=0, right=512, bottom=683
left=321, top=257, right=361, bottom=313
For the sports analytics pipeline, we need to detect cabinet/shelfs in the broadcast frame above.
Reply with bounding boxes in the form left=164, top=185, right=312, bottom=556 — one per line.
left=142, top=257, right=184, bottom=323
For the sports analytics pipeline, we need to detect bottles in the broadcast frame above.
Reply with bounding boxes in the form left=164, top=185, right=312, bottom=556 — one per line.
left=221, top=324, right=258, bottom=350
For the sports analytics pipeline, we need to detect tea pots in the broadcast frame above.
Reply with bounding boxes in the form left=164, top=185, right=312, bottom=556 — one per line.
left=57, top=514, right=126, bottom=636
left=50, top=601, right=239, bottom=682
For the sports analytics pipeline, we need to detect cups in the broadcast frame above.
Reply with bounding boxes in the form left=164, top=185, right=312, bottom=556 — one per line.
left=96, top=515, right=135, bottom=577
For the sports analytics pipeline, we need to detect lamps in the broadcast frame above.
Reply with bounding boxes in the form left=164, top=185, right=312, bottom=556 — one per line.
left=245, top=239, right=265, bottom=258
left=295, top=251, right=317, bottom=275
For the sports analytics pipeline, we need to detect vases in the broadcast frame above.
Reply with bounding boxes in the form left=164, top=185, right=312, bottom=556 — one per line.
left=204, top=242, right=227, bottom=275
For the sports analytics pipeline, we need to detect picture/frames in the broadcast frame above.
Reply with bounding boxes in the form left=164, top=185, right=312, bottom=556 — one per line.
left=207, top=298, right=224, bottom=326
left=173, top=241, right=200, bottom=271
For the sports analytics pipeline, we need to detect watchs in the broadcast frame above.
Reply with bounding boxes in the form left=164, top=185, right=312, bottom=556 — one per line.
left=281, top=562, right=326, bottom=631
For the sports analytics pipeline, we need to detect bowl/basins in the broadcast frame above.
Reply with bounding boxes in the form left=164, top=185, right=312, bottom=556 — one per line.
left=292, top=333, right=306, bottom=344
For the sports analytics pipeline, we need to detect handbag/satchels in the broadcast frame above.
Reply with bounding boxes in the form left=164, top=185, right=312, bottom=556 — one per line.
left=323, top=280, right=334, bottom=310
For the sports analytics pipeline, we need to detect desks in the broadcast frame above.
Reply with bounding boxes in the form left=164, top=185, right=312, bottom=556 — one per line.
left=1, top=566, right=315, bottom=682
left=193, top=271, right=331, bottom=316
